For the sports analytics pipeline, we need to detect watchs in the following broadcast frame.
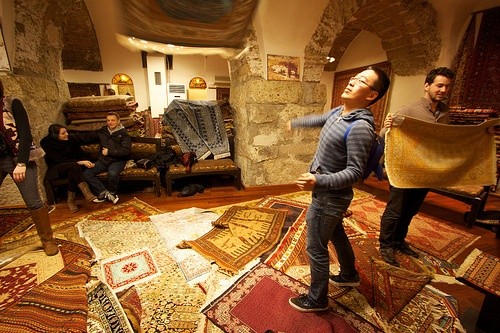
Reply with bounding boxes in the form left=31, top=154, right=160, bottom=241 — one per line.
left=18, top=163, right=26, bottom=166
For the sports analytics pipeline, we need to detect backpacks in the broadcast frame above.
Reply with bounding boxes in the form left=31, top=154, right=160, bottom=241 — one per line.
left=329, top=108, right=385, bottom=180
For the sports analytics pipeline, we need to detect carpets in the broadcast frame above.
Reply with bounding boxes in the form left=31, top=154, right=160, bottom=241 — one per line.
left=0, top=116, right=499, bottom=333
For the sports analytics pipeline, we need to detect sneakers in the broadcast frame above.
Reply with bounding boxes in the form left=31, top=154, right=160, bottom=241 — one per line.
left=93, top=196, right=106, bottom=202
left=289, top=293, right=328, bottom=312
left=328, top=275, right=360, bottom=286
left=105, top=192, right=119, bottom=204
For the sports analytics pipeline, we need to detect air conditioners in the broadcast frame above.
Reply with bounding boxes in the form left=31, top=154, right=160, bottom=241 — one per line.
left=167, top=83, right=187, bottom=105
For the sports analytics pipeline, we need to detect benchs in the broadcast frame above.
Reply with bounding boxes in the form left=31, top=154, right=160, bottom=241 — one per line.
left=430, top=185, right=489, bottom=227
left=45, top=136, right=160, bottom=201
left=165, top=138, right=241, bottom=195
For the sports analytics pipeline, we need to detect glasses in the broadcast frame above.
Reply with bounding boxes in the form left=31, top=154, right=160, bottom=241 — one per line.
left=350, top=76, right=376, bottom=91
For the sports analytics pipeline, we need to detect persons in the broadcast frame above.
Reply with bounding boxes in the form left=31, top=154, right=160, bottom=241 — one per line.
left=378, top=67, right=495, bottom=266
left=40, top=124, right=105, bottom=213
left=70, top=111, right=132, bottom=204
left=0, top=81, right=59, bottom=256
left=285, top=66, right=391, bottom=312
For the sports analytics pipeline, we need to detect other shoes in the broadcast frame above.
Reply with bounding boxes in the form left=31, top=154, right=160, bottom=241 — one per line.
left=380, top=248, right=395, bottom=264
left=397, top=244, right=416, bottom=255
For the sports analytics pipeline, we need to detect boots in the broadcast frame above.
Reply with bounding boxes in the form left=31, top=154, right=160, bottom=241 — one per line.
left=67, top=191, right=78, bottom=212
left=27, top=203, right=58, bottom=256
left=78, top=182, right=96, bottom=202
